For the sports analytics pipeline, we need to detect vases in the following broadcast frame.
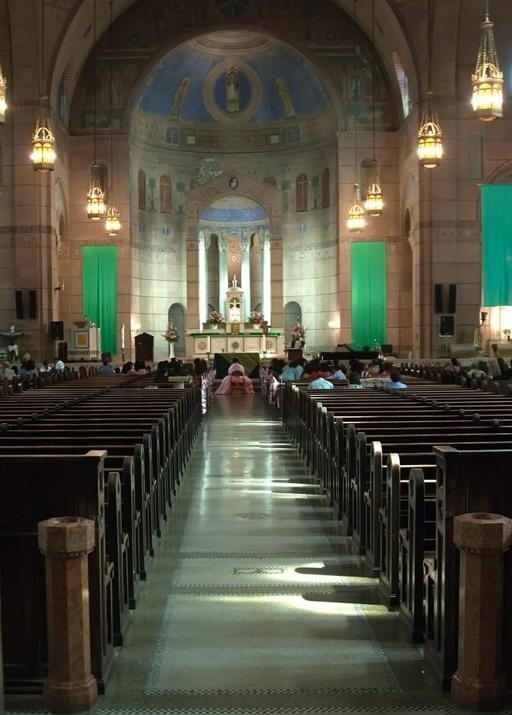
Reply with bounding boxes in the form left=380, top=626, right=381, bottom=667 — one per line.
left=211, top=323, right=218, bottom=331
left=169, top=341, right=175, bottom=359
left=253, top=324, right=262, bottom=329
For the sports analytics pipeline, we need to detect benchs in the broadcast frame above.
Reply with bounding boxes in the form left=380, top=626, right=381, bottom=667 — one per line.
left=1, top=369, right=213, bottom=695
left=282, top=362, right=512, bottom=715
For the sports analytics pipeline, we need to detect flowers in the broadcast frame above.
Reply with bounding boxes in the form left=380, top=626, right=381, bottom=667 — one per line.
left=287, top=324, right=307, bottom=341
left=208, top=310, right=223, bottom=323
left=249, top=311, right=264, bottom=324
left=161, top=318, right=179, bottom=342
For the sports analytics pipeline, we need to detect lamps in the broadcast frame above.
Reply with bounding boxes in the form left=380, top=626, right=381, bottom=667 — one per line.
left=85, top=0, right=123, bottom=238
left=346, top=0, right=386, bottom=236
left=470, top=1, right=505, bottom=123
left=30, top=0, right=58, bottom=173
left=416, top=1, right=445, bottom=169
